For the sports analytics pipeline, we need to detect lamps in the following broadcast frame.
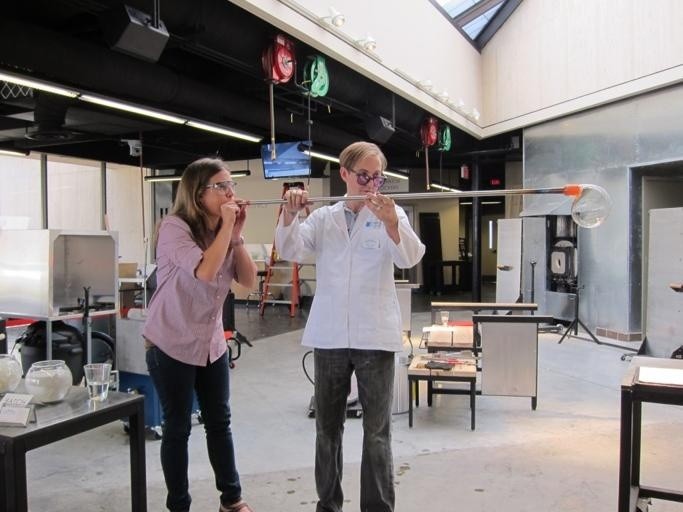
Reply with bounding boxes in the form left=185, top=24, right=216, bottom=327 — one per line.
left=356, top=36, right=377, bottom=50
left=319, top=5, right=345, bottom=26
left=417, top=79, right=480, bottom=120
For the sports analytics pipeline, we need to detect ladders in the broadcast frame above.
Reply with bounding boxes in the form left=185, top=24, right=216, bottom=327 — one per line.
left=259, top=181, right=305, bottom=317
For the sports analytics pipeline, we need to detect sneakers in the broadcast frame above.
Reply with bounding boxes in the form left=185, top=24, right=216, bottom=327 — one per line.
left=219, top=499, right=254, bottom=512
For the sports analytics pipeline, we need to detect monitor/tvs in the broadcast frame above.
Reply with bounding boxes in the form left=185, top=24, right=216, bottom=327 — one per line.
left=260, top=139, right=313, bottom=179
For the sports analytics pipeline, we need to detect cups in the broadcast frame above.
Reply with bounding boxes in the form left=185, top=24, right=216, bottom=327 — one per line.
left=440, top=311, right=450, bottom=324
left=82, top=363, right=112, bottom=402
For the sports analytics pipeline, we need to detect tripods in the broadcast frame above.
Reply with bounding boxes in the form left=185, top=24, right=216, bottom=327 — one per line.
left=558, top=288, right=600, bottom=345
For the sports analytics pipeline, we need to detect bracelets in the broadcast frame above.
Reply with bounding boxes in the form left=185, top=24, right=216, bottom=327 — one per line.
left=230, top=235, right=244, bottom=248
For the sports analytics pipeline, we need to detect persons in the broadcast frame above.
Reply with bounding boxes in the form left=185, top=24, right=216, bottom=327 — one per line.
left=274, top=142, right=426, bottom=511
left=139, top=157, right=256, bottom=512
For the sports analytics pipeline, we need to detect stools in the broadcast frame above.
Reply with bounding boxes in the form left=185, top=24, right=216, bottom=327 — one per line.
left=246, top=272, right=275, bottom=310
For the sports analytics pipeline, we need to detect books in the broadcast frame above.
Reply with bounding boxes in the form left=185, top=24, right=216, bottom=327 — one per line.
left=423, top=360, right=453, bottom=371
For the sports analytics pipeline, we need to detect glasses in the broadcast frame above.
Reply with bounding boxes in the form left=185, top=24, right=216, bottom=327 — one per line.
left=350, top=168, right=388, bottom=189
left=205, top=180, right=238, bottom=193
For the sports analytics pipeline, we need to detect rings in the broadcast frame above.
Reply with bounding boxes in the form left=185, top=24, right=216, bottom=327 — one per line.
left=376, top=206, right=380, bottom=210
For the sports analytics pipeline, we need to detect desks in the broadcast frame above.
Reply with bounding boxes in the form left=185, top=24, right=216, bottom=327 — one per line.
left=619, top=356, right=683, bottom=512
left=408, top=302, right=538, bottom=431
left=0, top=378, right=147, bottom=512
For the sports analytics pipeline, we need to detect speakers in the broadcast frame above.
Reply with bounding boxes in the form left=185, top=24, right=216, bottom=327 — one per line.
left=102, top=5, right=170, bottom=64
left=365, top=116, right=395, bottom=144
